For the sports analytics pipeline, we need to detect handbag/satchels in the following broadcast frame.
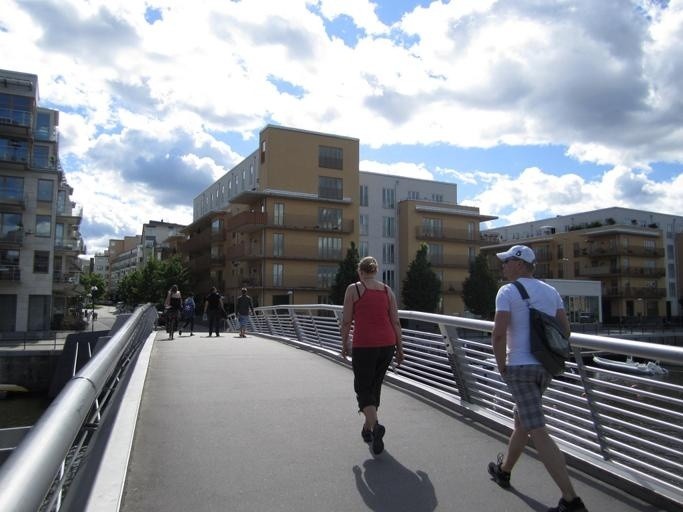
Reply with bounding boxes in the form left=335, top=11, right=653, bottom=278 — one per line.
left=512, top=280, right=570, bottom=377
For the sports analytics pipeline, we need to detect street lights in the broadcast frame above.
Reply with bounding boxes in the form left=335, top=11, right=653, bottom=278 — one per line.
left=90, top=284, right=99, bottom=331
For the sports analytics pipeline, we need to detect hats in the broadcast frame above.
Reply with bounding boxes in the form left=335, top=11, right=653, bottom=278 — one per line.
left=496, top=244, right=535, bottom=265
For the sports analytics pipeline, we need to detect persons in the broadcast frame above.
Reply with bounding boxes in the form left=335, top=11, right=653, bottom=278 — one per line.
left=487, top=242, right=591, bottom=511
left=235, top=287, right=256, bottom=338
left=203, top=286, right=224, bottom=337
left=339, top=256, right=404, bottom=454
left=179, top=292, right=198, bottom=336
left=164, top=284, right=183, bottom=330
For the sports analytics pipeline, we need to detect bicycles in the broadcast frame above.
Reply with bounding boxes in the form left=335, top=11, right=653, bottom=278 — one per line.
left=164, top=306, right=183, bottom=341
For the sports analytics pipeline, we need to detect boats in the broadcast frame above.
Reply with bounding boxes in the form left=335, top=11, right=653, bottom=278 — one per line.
left=593, top=355, right=669, bottom=376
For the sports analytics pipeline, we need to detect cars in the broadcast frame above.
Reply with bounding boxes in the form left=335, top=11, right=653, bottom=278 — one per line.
left=158, top=311, right=166, bottom=325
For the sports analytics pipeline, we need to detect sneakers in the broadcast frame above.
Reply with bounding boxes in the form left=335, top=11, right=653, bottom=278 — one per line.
left=240, top=335, right=246, bottom=338
left=209, top=334, right=219, bottom=337
left=361, top=424, right=373, bottom=443
left=179, top=330, right=195, bottom=336
left=371, top=424, right=386, bottom=455
left=548, top=497, right=588, bottom=512
left=487, top=461, right=511, bottom=488
